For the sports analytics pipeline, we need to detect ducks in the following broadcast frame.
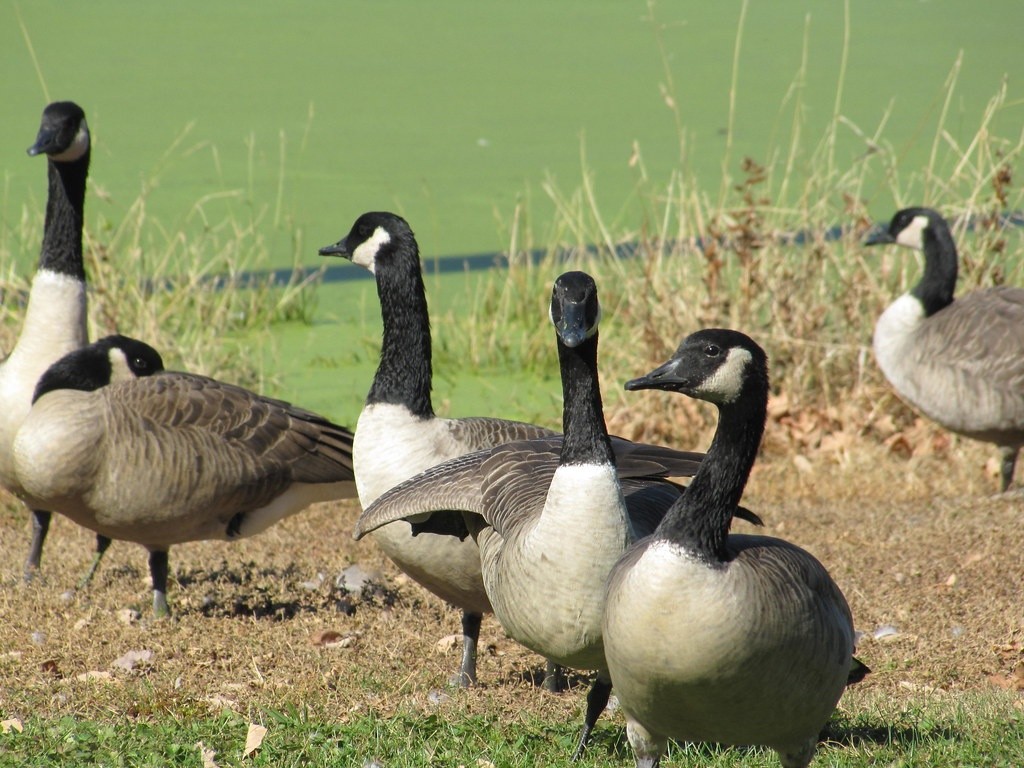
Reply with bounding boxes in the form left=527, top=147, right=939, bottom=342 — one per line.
left=0, top=100, right=1024, bottom=768
